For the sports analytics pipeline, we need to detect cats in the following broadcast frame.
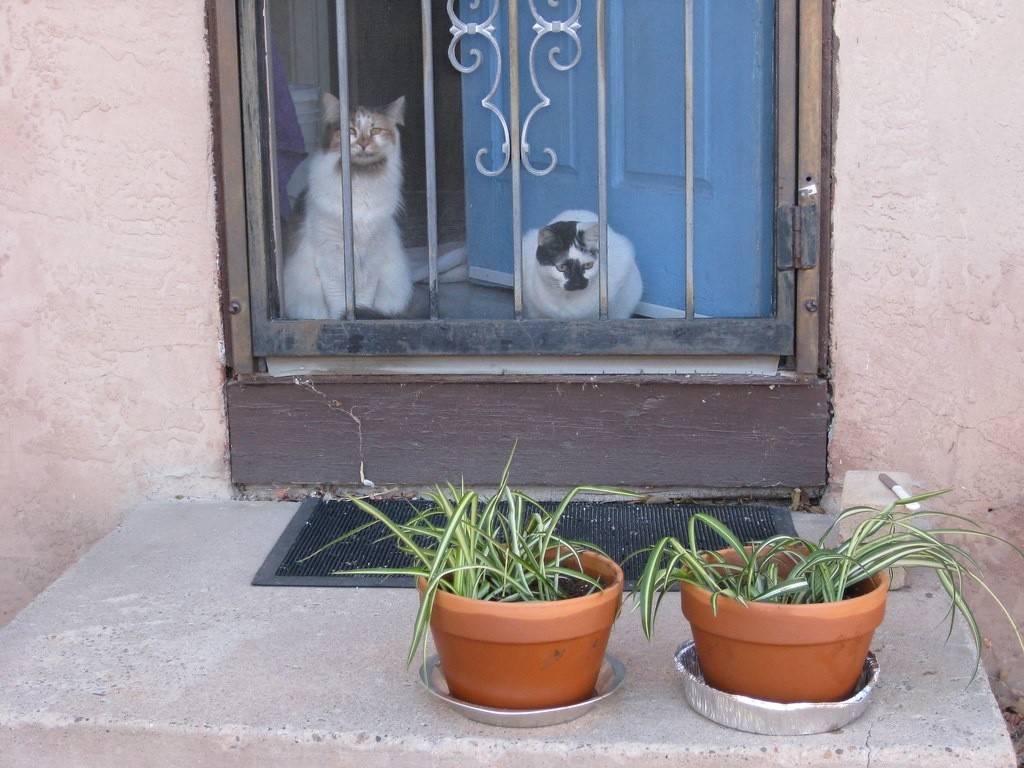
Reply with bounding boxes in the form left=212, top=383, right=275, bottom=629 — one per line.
left=520, top=211, right=644, bottom=319
left=283, top=92, right=414, bottom=319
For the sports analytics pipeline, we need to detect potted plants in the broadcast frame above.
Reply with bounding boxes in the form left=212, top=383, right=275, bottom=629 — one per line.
left=293, top=436, right=649, bottom=709
left=614, top=486, right=1024, bottom=702
